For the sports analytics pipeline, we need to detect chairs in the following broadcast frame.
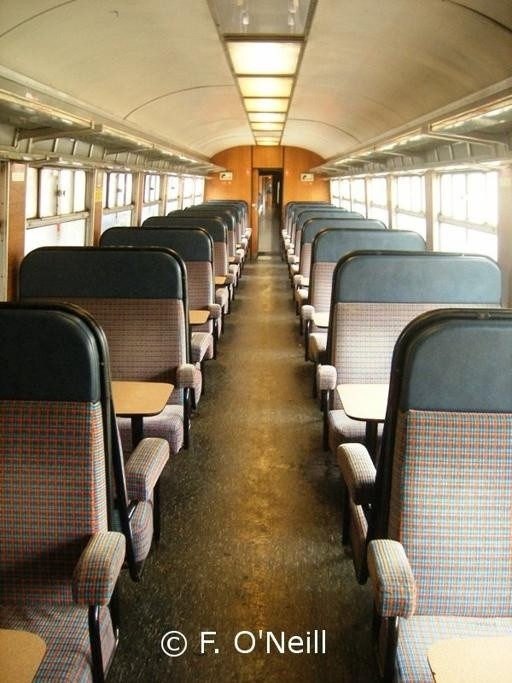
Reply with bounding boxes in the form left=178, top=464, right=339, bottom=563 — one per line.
left=281, top=200, right=512, bottom=683
left=1, top=200, right=253, bottom=683
